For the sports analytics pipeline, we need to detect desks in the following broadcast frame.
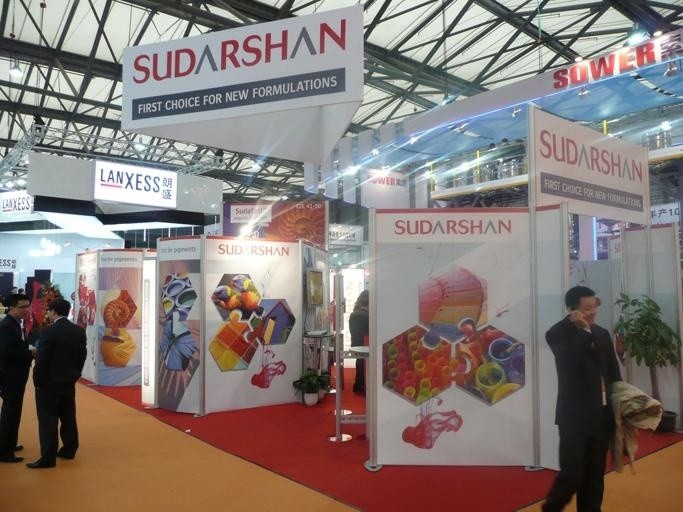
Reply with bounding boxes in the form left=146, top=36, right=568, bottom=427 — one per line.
left=306, top=331, right=335, bottom=375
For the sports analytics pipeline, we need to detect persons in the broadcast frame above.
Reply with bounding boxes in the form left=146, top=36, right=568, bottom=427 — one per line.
left=541, top=285, right=623, bottom=512
left=27, top=298, right=88, bottom=469
left=348, top=295, right=369, bottom=393
left=0, top=288, right=38, bottom=463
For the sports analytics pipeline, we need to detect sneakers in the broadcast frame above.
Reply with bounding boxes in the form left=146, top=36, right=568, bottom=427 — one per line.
left=56, top=447, right=74, bottom=460
left=12, top=445, right=24, bottom=452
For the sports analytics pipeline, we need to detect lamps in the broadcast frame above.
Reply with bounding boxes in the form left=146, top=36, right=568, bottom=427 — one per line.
left=513, top=105, right=522, bottom=117
left=455, top=123, right=469, bottom=133
left=578, top=86, right=591, bottom=98
left=664, top=63, right=679, bottom=77
left=10, top=60, right=22, bottom=76
left=135, top=137, right=144, bottom=149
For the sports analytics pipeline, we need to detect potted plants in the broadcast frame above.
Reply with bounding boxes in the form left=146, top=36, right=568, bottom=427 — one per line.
left=292, top=368, right=331, bottom=406
left=613, top=293, right=680, bottom=432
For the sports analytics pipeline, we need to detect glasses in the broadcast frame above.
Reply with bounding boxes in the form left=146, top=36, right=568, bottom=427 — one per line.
left=11, top=303, right=32, bottom=310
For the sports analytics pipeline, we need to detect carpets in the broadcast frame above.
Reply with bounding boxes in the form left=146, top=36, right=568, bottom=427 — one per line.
left=78, top=368, right=683, bottom=512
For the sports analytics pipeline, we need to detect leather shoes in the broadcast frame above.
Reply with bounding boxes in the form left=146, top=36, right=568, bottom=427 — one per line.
left=24, top=458, right=60, bottom=469
left=1, top=454, right=27, bottom=464
